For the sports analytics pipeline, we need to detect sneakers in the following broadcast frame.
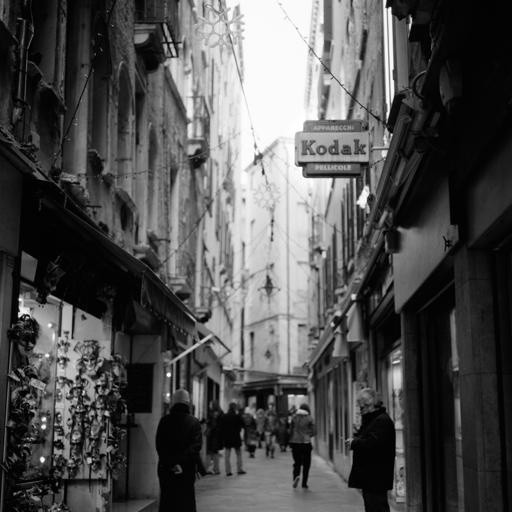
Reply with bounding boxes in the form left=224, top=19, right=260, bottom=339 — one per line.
left=292, top=475, right=300, bottom=488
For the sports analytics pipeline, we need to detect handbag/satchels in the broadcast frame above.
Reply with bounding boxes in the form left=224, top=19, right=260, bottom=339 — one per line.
left=284, top=418, right=294, bottom=447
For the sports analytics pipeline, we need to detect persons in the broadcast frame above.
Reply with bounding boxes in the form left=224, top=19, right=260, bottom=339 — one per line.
left=288, top=404, right=317, bottom=489
left=202, top=401, right=297, bottom=476
left=155, top=389, right=202, bottom=512
left=346, top=388, right=395, bottom=512
